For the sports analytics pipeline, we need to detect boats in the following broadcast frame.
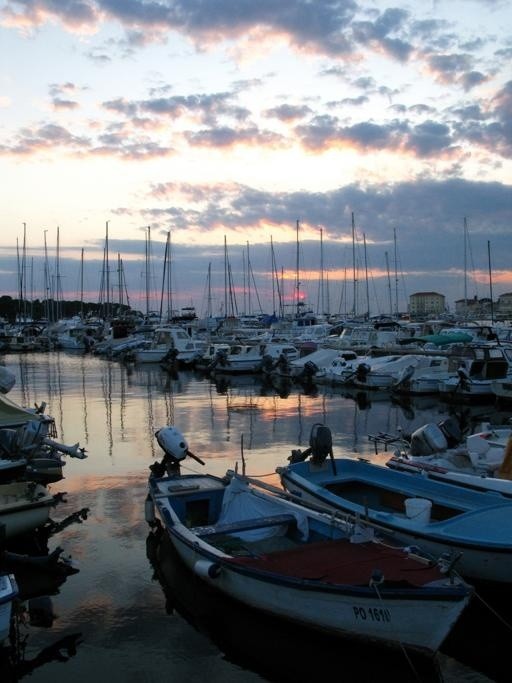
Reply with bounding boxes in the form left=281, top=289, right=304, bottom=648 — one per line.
left=145, top=426, right=512, bottom=683
left=144, top=425, right=474, bottom=661
left=0, top=477, right=56, bottom=537
left=333, top=210, right=512, bottom=404
left=0, top=401, right=90, bottom=475
left=0, top=366, right=91, bottom=660
left=0, top=222, right=176, bottom=365
left=176, top=218, right=334, bottom=382
